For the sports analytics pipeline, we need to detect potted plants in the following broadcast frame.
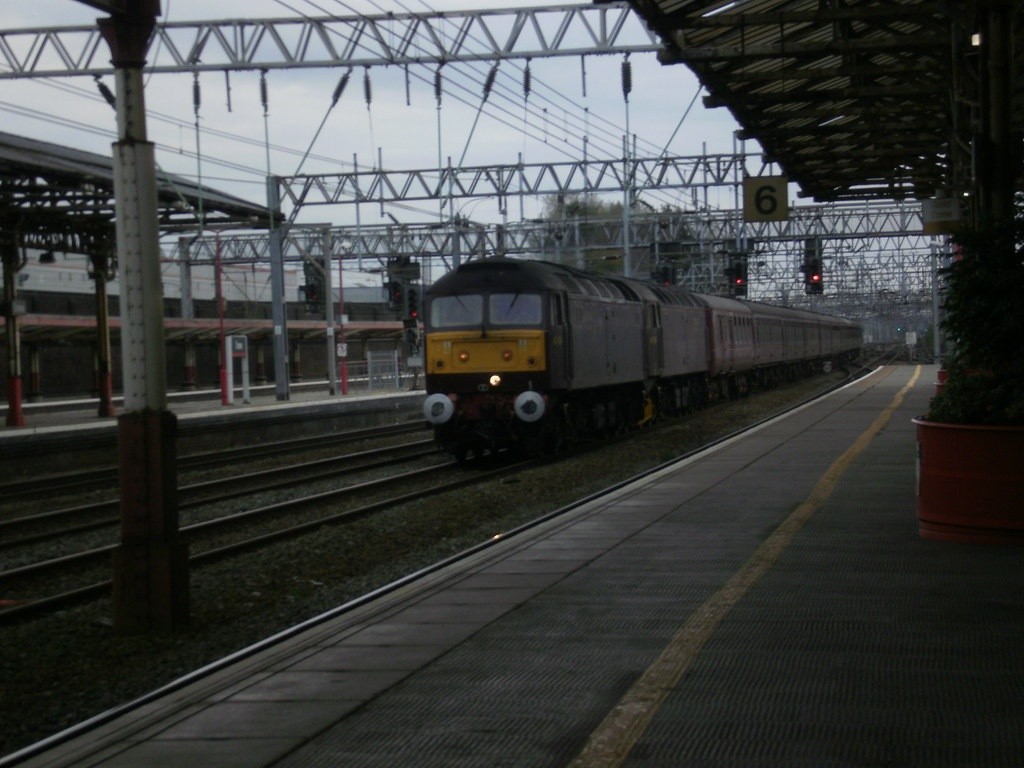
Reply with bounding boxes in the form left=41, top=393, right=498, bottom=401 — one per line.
left=911, top=179, right=1023, bottom=545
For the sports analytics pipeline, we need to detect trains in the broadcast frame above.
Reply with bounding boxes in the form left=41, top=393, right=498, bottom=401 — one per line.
left=417, top=255, right=866, bottom=454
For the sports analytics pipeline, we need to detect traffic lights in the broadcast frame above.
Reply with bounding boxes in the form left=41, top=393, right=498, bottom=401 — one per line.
left=732, top=262, right=746, bottom=295
left=804, top=260, right=823, bottom=296
left=405, top=286, right=419, bottom=321
left=390, top=284, right=404, bottom=311
